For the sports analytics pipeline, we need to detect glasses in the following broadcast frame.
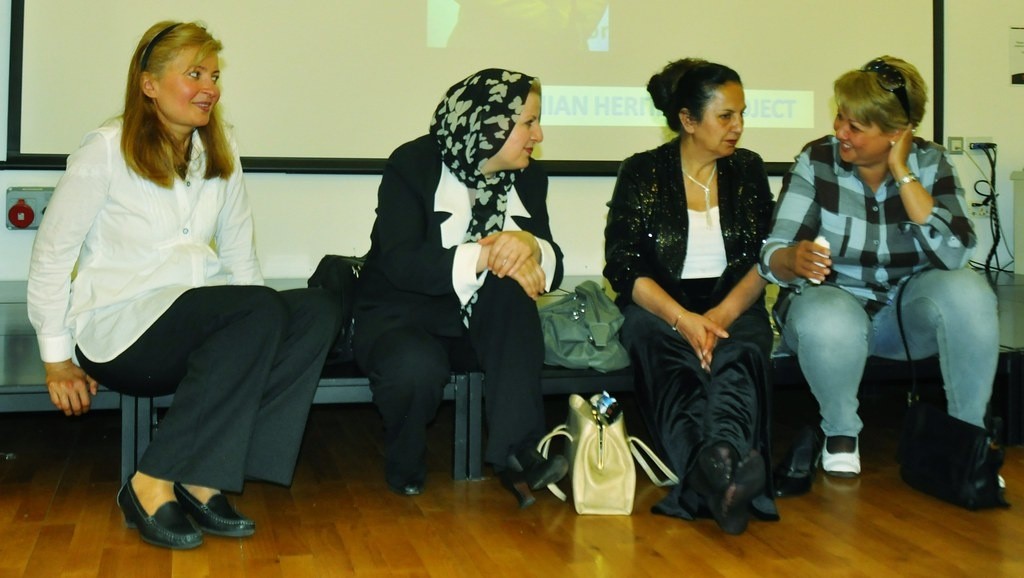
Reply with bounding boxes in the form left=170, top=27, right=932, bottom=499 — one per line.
left=859, top=56, right=918, bottom=124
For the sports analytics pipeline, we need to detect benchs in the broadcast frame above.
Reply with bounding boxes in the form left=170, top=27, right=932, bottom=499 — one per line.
left=0, top=162, right=1023, bottom=493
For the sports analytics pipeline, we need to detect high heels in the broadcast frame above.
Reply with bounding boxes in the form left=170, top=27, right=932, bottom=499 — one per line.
left=499, top=446, right=569, bottom=508
left=118, top=473, right=206, bottom=549
left=387, top=474, right=426, bottom=498
left=173, top=481, right=259, bottom=540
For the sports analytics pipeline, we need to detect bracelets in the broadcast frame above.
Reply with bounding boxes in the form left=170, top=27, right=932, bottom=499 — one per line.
left=895, top=173, right=919, bottom=190
left=672, top=309, right=689, bottom=331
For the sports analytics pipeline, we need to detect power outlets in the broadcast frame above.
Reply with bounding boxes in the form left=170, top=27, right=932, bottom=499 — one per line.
left=966, top=137, right=995, bottom=155
left=948, top=137, right=963, bottom=155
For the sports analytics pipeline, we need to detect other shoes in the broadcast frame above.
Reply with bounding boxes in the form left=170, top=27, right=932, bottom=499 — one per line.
left=819, top=432, right=864, bottom=479
left=774, top=423, right=826, bottom=497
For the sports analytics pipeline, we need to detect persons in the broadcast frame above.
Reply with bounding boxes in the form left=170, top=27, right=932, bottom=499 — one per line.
left=756, top=55, right=1007, bottom=489
left=603, top=57, right=776, bottom=534
left=352, top=70, right=563, bottom=512
left=25, top=20, right=344, bottom=549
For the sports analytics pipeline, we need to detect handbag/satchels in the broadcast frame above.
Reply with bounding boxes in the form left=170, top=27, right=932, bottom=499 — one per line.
left=900, top=395, right=1012, bottom=510
left=541, top=276, right=636, bottom=374
left=309, top=252, right=372, bottom=371
left=537, top=392, right=681, bottom=517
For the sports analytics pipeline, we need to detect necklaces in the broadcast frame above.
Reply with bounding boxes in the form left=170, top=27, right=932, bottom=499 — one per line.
left=681, top=162, right=717, bottom=228
